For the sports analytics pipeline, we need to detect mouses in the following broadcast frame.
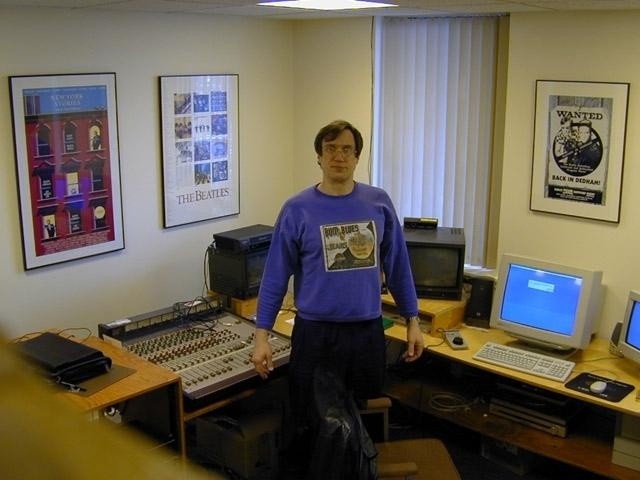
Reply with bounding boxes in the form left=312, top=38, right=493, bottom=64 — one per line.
left=590, top=381, right=607, bottom=394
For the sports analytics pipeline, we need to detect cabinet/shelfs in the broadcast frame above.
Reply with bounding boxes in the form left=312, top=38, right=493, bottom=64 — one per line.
left=12, top=332, right=184, bottom=480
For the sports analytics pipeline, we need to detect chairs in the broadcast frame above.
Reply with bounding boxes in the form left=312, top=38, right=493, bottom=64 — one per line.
left=306, top=367, right=458, bottom=479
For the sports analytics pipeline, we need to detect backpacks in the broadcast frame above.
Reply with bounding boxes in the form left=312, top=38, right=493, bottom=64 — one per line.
left=276, top=369, right=379, bottom=480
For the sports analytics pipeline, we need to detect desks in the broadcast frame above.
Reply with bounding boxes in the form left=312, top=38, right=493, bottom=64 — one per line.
left=235, top=290, right=639, bottom=480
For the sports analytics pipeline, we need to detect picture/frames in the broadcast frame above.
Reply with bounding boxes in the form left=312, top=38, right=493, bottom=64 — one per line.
left=529, top=80, right=630, bottom=224
left=158, top=75, right=241, bottom=229
left=9, top=72, right=124, bottom=271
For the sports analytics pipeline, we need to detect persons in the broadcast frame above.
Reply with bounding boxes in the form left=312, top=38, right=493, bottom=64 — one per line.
left=556, top=118, right=603, bottom=175
left=251, top=120, right=423, bottom=443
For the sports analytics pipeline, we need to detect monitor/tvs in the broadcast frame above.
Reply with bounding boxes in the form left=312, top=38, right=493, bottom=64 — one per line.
left=488, top=253, right=603, bottom=359
left=399, top=226, right=466, bottom=300
left=616, top=290, right=640, bottom=365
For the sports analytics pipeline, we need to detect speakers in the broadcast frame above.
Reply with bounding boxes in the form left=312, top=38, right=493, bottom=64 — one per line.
left=608, top=321, right=624, bottom=357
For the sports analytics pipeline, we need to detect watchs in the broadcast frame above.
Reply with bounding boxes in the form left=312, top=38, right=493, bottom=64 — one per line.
left=406, top=316, right=421, bottom=323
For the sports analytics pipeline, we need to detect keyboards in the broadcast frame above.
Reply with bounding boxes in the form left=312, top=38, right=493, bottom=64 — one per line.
left=472, top=341, right=575, bottom=383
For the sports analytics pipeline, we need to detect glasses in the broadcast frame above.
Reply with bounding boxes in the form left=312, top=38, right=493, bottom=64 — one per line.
left=321, top=144, right=360, bottom=157
